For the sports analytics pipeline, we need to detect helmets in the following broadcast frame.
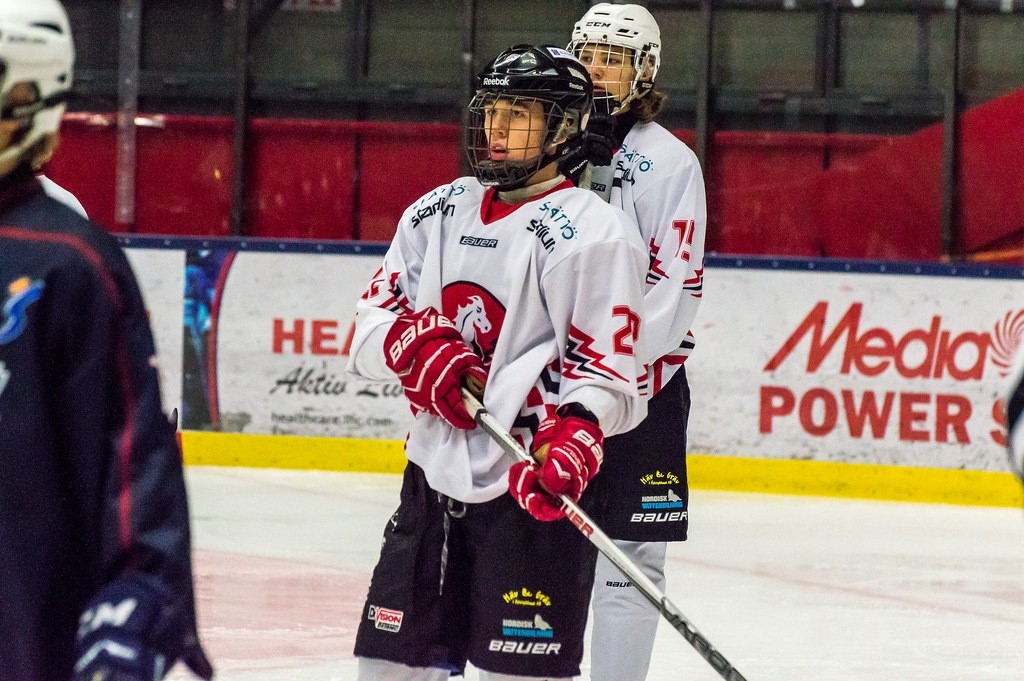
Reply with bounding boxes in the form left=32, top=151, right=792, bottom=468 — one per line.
left=473, top=43, right=594, bottom=192
left=0, top=0, right=76, bottom=185
left=572, top=2, right=661, bottom=116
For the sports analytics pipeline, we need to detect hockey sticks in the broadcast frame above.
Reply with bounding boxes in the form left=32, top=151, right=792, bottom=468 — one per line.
left=463, top=388, right=752, bottom=681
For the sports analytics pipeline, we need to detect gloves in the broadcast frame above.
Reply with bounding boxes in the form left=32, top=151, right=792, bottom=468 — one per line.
left=75, top=585, right=182, bottom=681
left=507, top=414, right=605, bottom=522
left=385, top=315, right=488, bottom=430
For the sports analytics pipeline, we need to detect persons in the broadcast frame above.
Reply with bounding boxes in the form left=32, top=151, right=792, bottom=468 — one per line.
left=1002, top=341, right=1024, bottom=481
left=550, top=1, right=707, bottom=681
left=0, top=0, right=215, bottom=681
left=346, top=41, right=648, bottom=681
